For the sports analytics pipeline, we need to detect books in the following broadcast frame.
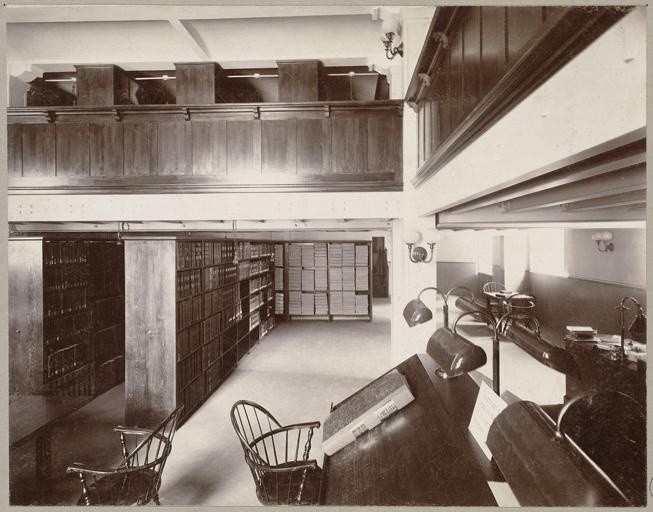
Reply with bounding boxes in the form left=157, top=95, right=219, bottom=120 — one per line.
left=177, top=334, right=223, bottom=392
left=314, top=292, right=328, bottom=315
left=177, top=283, right=237, bottom=332
left=178, top=358, right=221, bottom=421
left=237, top=240, right=274, bottom=260
left=328, top=267, right=342, bottom=290
left=288, top=243, right=302, bottom=267
left=249, top=310, right=262, bottom=332
left=274, top=244, right=284, bottom=266
left=320, top=368, right=416, bottom=457
left=314, top=269, right=327, bottom=291
left=288, top=291, right=302, bottom=315
left=239, top=256, right=270, bottom=281
left=301, top=243, right=314, bottom=267
left=176, top=263, right=238, bottom=301
left=258, top=313, right=274, bottom=339
left=343, top=243, right=355, bottom=266
left=176, top=240, right=235, bottom=270
left=356, top=266, right=369, bottom=290
left=355, top=294, right=369, bottom=315
left=302, top=269, right=314, bottom=291
left=288, top=268, right=301, bottom=290
left=274, top=267, right=284, bottom=290
left=302, top=292, right=314, bottom=315
left=314, top=243, right=328, bottom=267
left=249, top=286, right=273, bottom=312
left=330, top=291, right=343, bottom=315
left=342, top=267, right=355, bottom=290
left=328, top=242, right=343, bottom=266
left=249, top=271, right=272, bottom=293
left=176, top=300, right=243, bottom=363
left=355, top=245, right=369, bottom=266
left=343, top=290, right=356, bottom=315
left=275, top=292, right=284, bottom=315
left=44, top=240, right=125, bottom=397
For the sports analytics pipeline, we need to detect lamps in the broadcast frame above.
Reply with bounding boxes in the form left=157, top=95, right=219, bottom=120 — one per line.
left=403, top=228, right=438, bottom=264
left=379, top=17, right=404, bottom=60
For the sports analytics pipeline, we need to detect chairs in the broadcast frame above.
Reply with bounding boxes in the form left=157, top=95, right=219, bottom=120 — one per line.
left=66, top=404, right=184, bottom=506
left=231, top=400, right=322, bottom=506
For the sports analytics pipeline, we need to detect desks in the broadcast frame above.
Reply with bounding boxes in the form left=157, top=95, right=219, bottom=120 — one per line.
left=9, top=396, right=93, bottom=504
